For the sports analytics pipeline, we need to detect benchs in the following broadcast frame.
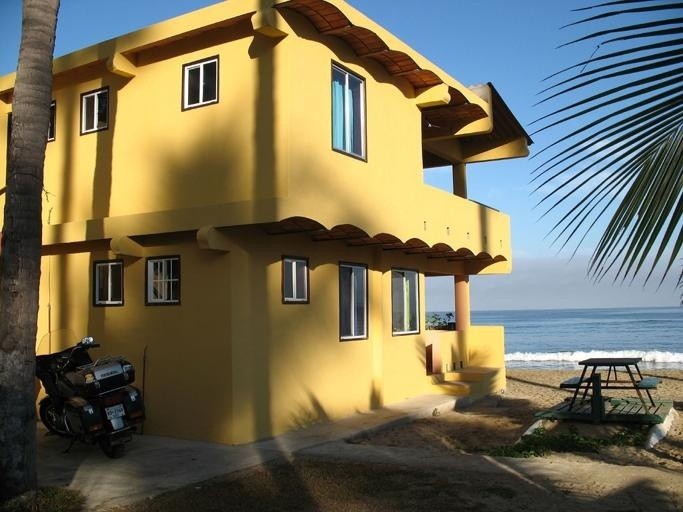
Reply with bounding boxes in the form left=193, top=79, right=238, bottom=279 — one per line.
left=639, top=376, right=660, bottom=413
left=559, top=377, right=590, bottom=410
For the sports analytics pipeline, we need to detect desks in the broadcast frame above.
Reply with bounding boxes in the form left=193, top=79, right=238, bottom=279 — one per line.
left=569, top=358, right=655, bottom=414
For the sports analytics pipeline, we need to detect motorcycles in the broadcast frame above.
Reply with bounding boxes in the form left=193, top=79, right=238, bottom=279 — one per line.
left=36, top=337, right=146, bottom=459
